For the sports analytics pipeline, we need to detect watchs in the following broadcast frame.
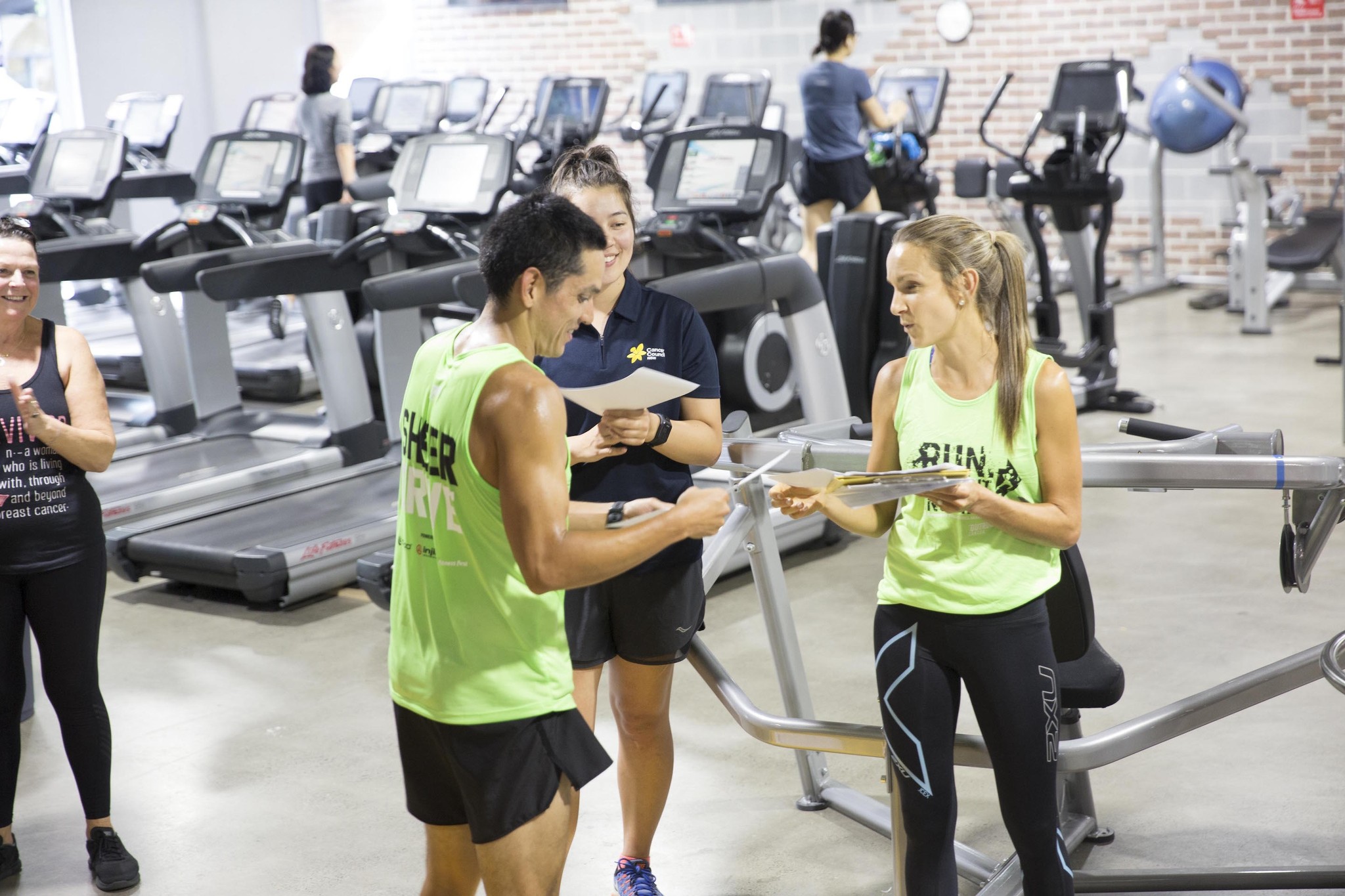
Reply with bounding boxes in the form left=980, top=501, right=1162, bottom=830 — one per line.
left=645, top=413, right=672, bottom=447
left=606, top=500, right=627, bottom=523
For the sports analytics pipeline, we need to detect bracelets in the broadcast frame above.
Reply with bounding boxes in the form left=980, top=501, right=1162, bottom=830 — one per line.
left=343, top=184, right=348, bottom=190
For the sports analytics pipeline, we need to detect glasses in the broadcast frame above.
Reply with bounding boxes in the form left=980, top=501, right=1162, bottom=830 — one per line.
left=0, top=217, right=32, bottom=229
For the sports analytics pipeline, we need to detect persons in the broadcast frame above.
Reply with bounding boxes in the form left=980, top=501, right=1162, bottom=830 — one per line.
left=290, top=43, right=358, bottom=215
left=802, top=8, right=910, bottom=274
left=768, top=215, right=1082, bottom=896
left=533, top=146, right=724, bottom=896
left=0, top=213, right=141, bottom=892
left=389, top=191, right=730, bottom=896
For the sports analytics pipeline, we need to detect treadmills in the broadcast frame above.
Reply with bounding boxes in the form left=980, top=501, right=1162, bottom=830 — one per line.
left=0, top=47, right=1147, bottom=611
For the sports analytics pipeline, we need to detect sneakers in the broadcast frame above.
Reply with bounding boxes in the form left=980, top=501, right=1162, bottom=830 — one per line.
left=86, top=827, right=140, bottom=891
left=614, top=858, right=664, bottom=896
left=0, top=833, right=21, bottom=878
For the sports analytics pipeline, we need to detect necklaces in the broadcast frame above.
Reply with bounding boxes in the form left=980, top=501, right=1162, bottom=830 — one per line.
left=0, top=317, right=27, bottom=366
